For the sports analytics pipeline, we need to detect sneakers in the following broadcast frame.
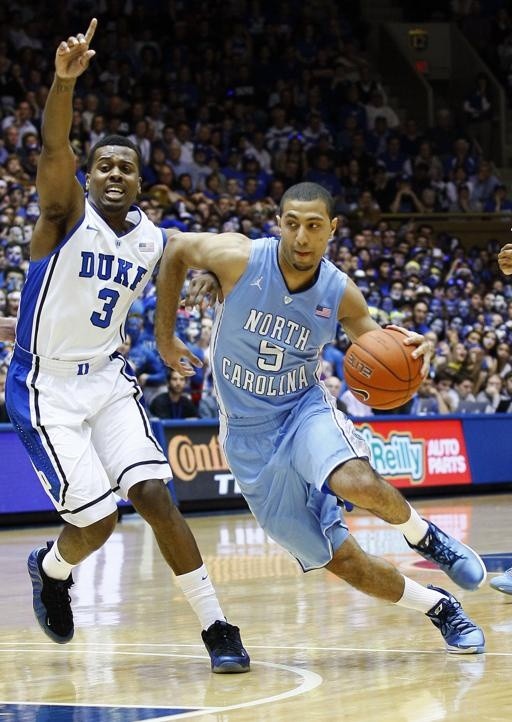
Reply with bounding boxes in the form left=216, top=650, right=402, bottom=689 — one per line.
left=199, top=619, right=251, bottom=673
left=402, top=513, right=488, bottom=593
left=422, top=582, right=486, bottom=655
left=25, top=538, right=77, bottom=645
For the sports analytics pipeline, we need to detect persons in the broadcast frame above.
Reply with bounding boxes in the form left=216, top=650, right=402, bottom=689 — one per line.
left=7, top=17, right=250, bottom=674
left=491, top=244, right=511, bottom=594
left=158, top=182, right=488, bottom=651
left=0, top=0, right=511, bottom=420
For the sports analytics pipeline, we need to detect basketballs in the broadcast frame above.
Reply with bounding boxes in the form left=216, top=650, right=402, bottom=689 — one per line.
left=343, top=331, right=425, bottom=409
